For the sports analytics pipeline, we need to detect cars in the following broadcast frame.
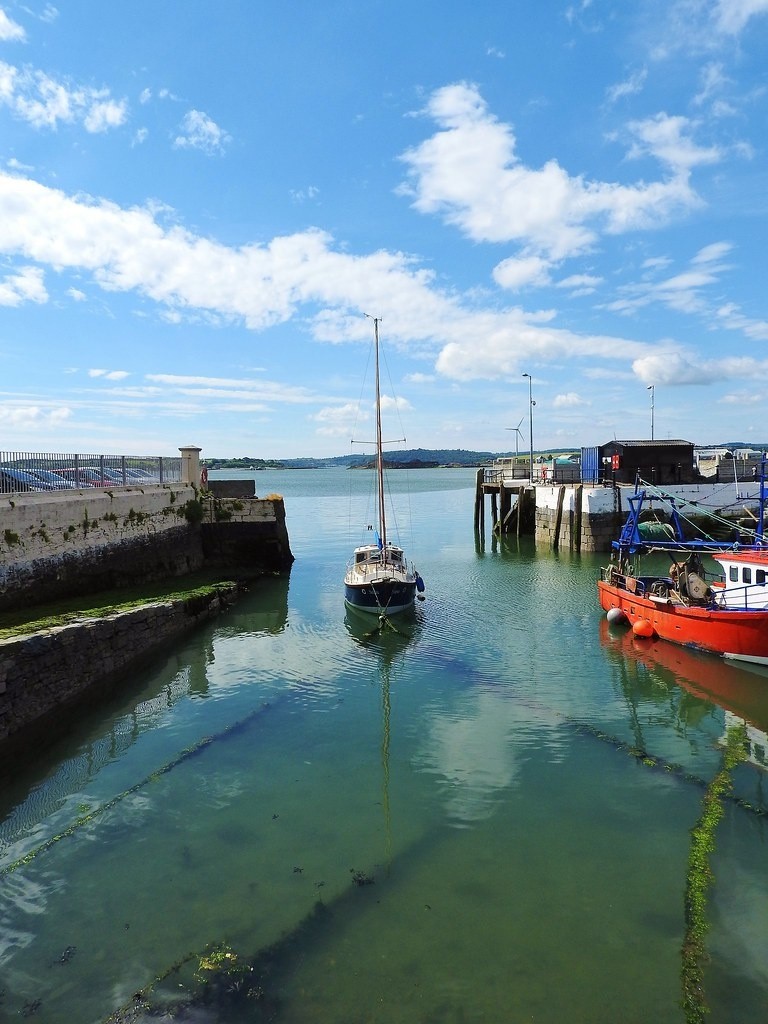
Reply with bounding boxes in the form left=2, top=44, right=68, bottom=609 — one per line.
left=0, top=467, right=160, bottom=493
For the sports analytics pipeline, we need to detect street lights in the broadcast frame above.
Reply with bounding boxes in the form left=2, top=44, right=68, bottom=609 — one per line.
left=647, top=384, right=654, bottom=440
left=522, top=374, right=536, bottom=483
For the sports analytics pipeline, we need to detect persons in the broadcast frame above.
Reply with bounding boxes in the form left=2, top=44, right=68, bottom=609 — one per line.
left=751, top=465, right=758, bottom=482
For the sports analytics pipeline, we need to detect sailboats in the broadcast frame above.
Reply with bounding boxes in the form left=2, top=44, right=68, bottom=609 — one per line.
left=343, top=313, right=416, bottom=615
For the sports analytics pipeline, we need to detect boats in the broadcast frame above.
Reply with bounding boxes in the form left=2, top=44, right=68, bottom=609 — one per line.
left=596, top=449, right=768, bottom=666
left=599, top=612, right=768, bottom=775
